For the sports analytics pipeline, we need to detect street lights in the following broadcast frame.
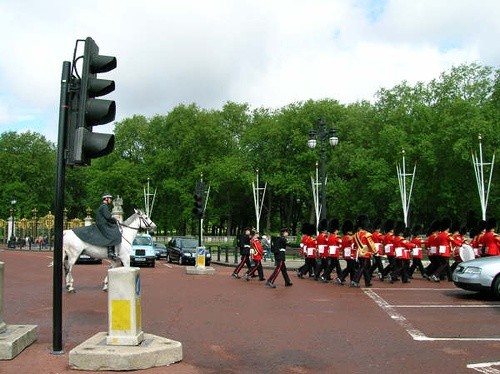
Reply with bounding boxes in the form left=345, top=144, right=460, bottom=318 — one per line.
left=9, top=200, right=18, bottom=248
left=307, top=117, right=339, bottom=221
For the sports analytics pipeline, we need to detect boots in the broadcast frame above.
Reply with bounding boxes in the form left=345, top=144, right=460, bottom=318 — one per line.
left=107, top=247, right=117, bottom=260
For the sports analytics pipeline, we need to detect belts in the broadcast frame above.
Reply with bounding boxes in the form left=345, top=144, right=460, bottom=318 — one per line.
left=279, top=248, right=286, bottom=252
left=245, top=245, right=251, bottom=248
left=300, top=243, right=447, bottom=250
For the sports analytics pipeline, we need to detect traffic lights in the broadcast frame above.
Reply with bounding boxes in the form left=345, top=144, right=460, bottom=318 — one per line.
left=73, top=35, right=118, bottom=166
left=192, top=193, right=203, bottom=217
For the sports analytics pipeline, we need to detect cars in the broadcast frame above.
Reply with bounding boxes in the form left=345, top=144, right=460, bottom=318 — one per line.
left=452, top=254, right=500, bottom=297
left=153, top=242, right=168, bottom=259
left=76, top=250, right=103, bottom=264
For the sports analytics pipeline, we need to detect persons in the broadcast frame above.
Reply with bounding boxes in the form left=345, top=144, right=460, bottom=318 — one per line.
left=232, top=228, right=258, bottom=278
left=265, top=229, right=304, bottom=288
left=243, top=230, right=266, bottom=281
left=263, top=233, right=274, bottom=262
left=16, top=235, right=48, bottom=250
left=297, top=214, right=500, bottom=288
left=96, top=194, right=119, bottom=258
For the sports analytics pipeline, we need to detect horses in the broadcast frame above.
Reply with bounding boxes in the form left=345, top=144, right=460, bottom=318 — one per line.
left=47, top=207, right=158, bottom=294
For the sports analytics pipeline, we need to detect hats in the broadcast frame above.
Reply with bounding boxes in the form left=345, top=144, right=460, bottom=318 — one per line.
left=281, top=227, right=289, bottom=231
left=301, top=214, right=500, bottom=239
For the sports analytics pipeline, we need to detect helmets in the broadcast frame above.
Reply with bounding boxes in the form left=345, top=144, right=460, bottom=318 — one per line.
left=101, top=192, right=112, bottom=198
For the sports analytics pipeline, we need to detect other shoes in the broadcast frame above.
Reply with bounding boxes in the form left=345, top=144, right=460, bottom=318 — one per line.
left=296, top=271, right=453, bottom=288
left=244, top=273, right=249, bottom=279
left=232, top=273, right=239, bottom=278
left=259, top=278, right=265, bottom=281
left=286, top=282, right=293, bottom=286
left=251, top=274, right=257, bottom=278
left=267, top=281, right=278, bottom=288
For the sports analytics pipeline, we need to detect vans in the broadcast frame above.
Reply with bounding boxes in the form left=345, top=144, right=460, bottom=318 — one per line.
left=121, top=233, right=157, bottom=268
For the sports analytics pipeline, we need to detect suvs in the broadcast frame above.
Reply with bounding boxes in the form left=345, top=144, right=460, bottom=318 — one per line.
left=166, top=236, right=211, bottom=266
